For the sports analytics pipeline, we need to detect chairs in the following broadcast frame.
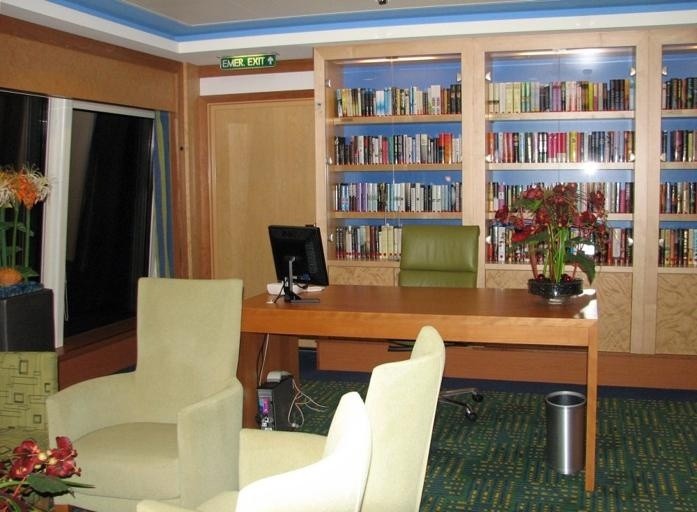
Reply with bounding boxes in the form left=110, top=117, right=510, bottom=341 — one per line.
left=387, top=225, right=484, bottom=423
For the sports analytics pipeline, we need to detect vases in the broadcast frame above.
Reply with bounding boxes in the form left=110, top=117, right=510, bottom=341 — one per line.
left=495, top=181, right=609, bottom=283
left=1, top=288, right=54, bottom=352
left=0, top=165, right=52, bottom=296
left=1, top=435, right=96, bottom=512
left=529, top=276, right=584, bottom=298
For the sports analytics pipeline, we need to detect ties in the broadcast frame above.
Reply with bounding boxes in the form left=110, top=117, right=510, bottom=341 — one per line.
left=268, top=225, right=329, bottom=303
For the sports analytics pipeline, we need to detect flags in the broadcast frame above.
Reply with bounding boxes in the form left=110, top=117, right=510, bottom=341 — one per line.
left=1, top=288, right=54, bottom=352
left=529, top=276, right=584, bottom=298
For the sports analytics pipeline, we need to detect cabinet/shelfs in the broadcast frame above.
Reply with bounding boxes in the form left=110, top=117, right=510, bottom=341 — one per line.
left=317, top=23, right=697, bottom=389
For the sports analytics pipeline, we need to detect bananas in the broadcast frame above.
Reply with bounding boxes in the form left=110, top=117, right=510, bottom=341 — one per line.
left=302, top=286, right=324, bottom=292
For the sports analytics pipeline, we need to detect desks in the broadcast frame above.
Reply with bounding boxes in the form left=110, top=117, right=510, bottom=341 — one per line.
left=235, top=284, right=599, bottom=493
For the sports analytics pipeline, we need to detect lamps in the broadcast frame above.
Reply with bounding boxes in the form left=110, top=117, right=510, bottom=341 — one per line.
left=387, top=225, right=484, bottom=423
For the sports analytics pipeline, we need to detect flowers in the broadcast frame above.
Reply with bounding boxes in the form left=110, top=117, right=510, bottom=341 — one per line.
left=495, top=181, right=609, bottom=283
left=0, top=165, right=52, bottom=296
left=1, top=435, right=96, bottom=512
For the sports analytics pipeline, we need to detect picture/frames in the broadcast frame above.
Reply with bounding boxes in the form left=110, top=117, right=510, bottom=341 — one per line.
left=235, top=284, right=599, bottom=493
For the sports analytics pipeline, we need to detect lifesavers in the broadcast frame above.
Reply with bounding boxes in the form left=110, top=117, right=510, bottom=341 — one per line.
left=256, top=375, right=294, bottom=431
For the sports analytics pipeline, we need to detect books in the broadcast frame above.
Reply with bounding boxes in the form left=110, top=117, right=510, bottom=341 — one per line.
left=661, top=77, right=695, bottom=110
left=658, top=228, right=696, bottom=266
left=336, top=223, right=402, bottom=260
left=334, top=131, right=462, bottom=165
left=661, top=181, right=695, bottom=214
left=488, top=225, right=633, bottom=266
left=488, top=130, right=634, bottom=164
left=335, top=181, right=462, bottom=212
left=488, top=68, right=635, bottom=115
left=337, top=84, right=461, bottom=117
left=662, top=129, right=695, bottom=162
left=488, top=181, right=633, bottom=213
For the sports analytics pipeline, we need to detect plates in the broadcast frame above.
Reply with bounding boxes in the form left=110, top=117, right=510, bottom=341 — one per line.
left=317, top=23, right=697, bottom=389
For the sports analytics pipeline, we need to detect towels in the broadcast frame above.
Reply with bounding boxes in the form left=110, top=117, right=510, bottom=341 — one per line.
left=545, top=391, right=586, bottom=474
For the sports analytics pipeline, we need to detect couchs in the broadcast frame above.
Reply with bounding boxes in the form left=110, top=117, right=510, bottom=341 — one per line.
left=137, top=326, right=445, bottom=512
left=47, top=276, right=244, bottom=510
